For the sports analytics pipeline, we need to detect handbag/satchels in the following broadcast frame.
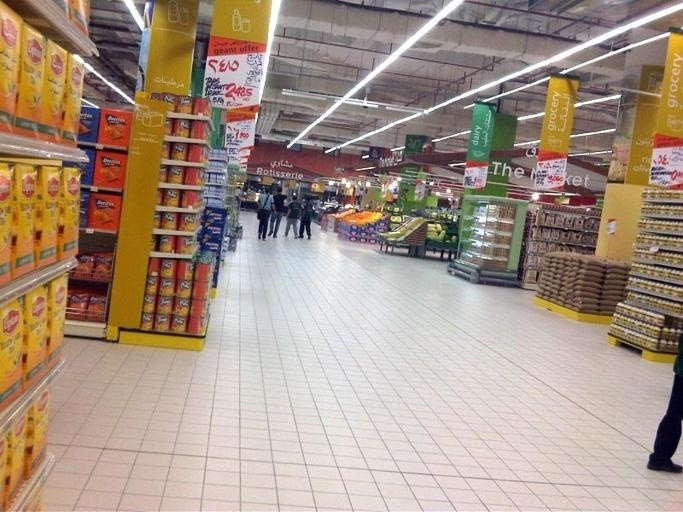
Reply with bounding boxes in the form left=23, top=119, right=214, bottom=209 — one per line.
left=256, top=209, right=269, bottom=219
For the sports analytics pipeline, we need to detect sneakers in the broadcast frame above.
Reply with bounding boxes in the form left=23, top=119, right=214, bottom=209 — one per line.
left=259, top=232, right=311, bottom=240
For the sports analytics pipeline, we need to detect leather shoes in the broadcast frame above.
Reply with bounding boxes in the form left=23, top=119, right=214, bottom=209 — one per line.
left=646, top=458, right=683, bottom=471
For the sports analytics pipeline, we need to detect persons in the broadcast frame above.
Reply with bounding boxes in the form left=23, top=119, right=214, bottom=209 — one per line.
left=257, top=187, right=314, bottom=242
left=234, top=187, right=240, bottom=209
left=647, top=331, right=683, bottom=472
